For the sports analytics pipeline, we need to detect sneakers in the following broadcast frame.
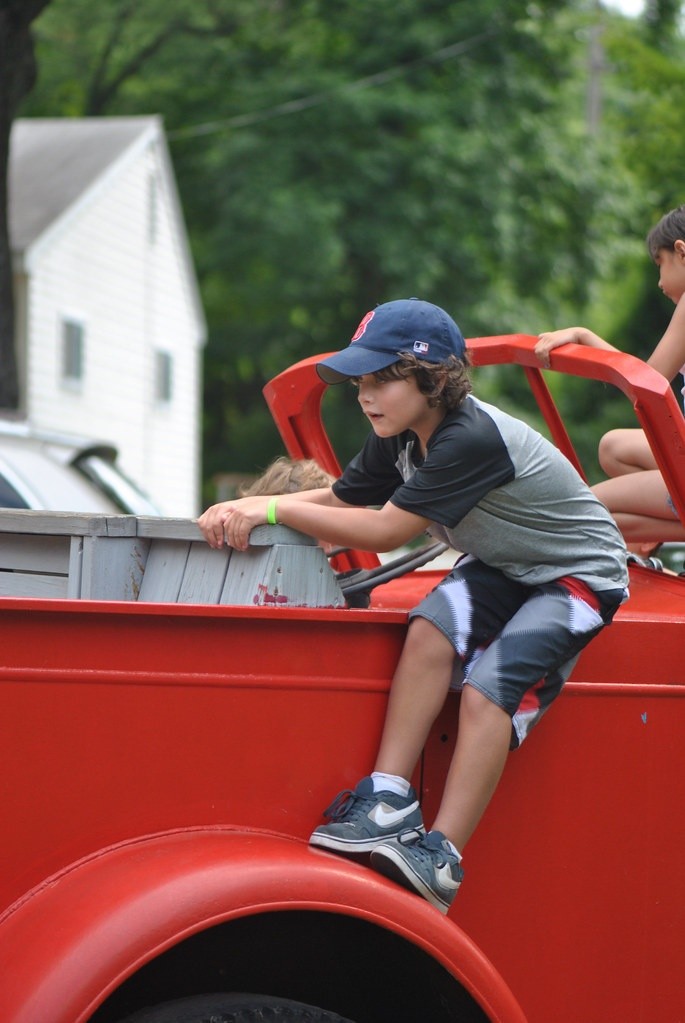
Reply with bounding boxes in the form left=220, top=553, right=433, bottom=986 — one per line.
left=370, top=828, right=464, bottom=915
left=308, top=776, right=426, bottom=852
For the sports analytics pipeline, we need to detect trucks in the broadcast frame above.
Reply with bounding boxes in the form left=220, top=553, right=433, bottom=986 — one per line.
left=0, top=328, right=685, bottom=1022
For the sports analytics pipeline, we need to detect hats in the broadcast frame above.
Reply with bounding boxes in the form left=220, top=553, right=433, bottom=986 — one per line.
left=315, top=297, right=469, bottom=385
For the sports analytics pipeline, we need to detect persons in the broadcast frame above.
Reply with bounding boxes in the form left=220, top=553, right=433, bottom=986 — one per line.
left=237, top=455, right=338, bottom=579
left=198, top=296, right=630, bottom=916
left=625, top=538, right=685, bottom=578
left=533, top=205, right=684, bottom=542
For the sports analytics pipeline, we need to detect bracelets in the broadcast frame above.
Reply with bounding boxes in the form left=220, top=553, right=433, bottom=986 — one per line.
left=267, top=499, right=277, bottom=525
